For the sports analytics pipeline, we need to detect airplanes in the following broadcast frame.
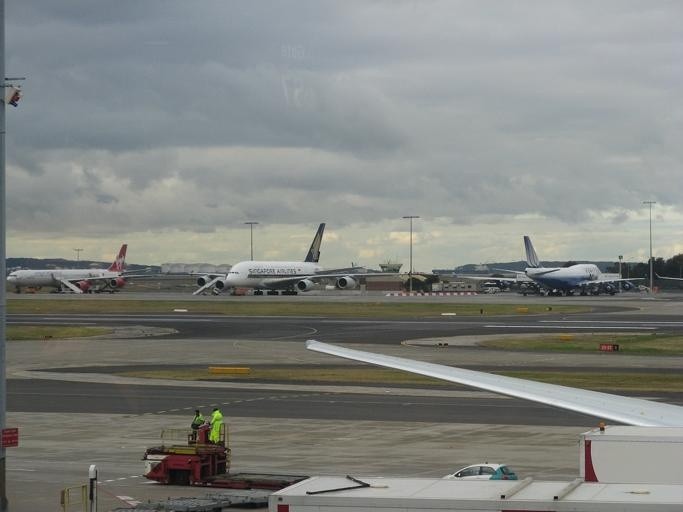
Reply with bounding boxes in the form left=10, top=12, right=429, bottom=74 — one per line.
left=456, top=234, right=644, bottom=301
left=305, top=339, right=682, bottom=430
left=4, top=243, right=153, bottom=296
left=189, top=222, right=402, bottom=297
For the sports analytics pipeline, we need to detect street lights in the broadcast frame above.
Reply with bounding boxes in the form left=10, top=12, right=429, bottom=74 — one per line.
left=243, top=221, right=258, bottom=260
left=400, top=215, right=420, bottom=293
left=642, top=200, right=656, bottom=295
left=72, top=247, right=83, bottom=266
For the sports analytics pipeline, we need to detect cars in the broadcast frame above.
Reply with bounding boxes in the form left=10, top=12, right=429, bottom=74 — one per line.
left=441, top=461, right=517, bottom=482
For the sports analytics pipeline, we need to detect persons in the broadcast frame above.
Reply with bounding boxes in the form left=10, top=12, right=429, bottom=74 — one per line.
left=190, top=408, right=205, bottom=430
left=208, top=406, right=224, bottom=445
